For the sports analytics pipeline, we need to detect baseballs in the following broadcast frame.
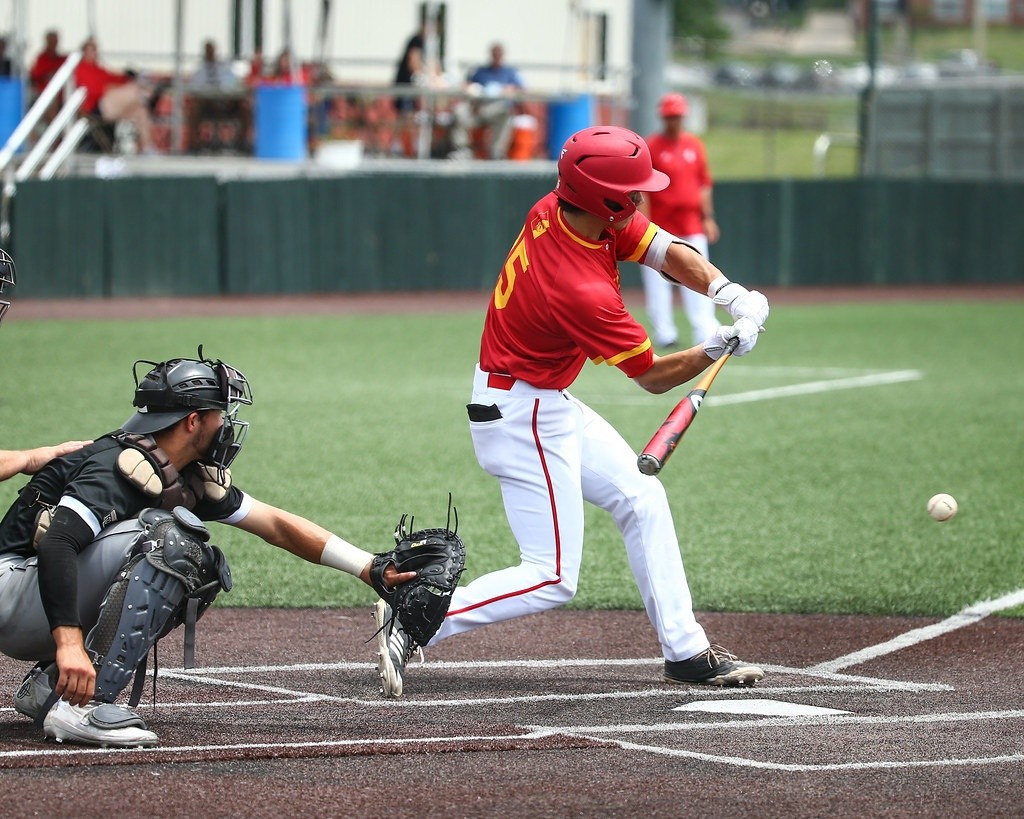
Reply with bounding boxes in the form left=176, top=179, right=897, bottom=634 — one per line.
left=926, top=493, right=958, bottom=523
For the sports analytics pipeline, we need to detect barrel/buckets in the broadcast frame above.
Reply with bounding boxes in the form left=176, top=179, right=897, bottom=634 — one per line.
left=256, top=85, right=309, bottom=161
left=0, top=77, right=24, bottom=154
left=549, top=93, right=593, bottom=160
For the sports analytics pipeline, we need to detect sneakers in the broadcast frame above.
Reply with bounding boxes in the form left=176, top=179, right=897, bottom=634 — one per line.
left=12, top=668, right=52, bottom=719
left=43, top=695, right=160, bottom=749
left=369, top=598, right=424, bottom=696
left=663, top=643, right=765, bottom=685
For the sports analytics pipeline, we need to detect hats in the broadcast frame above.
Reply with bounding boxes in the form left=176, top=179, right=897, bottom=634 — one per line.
left=658, top=94, right=687, bottom=117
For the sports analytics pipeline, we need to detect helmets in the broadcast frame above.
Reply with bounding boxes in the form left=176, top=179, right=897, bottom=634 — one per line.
left=552, top=125, right=671, bottom=222
left=122, top=359, right=224, bottom=434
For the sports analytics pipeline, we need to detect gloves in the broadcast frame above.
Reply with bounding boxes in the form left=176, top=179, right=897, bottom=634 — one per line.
left=703, top=316, right=759, bottom=360
left=707, top=276, right=770, bottom=333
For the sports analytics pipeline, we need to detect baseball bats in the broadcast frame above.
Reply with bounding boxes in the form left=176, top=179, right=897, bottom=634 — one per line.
left=635, top=337, right=739, bottom=475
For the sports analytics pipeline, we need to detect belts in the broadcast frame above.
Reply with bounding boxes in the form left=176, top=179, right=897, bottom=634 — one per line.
left=487, top=374, right=516, bottom=390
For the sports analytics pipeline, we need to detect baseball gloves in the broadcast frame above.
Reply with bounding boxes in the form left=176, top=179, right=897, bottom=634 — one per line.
left=370, top=527, right=467, bottom=648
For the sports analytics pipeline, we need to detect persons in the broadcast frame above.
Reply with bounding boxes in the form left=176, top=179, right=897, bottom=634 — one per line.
left=636, top=93, right=723, bottom=347
left=393, top=11, right=441, bottom=117
left=468, top=42, right=527, bottom=118
left=375, top=126, right=770, bottom=696
left=1, top=249, right=417, bottom=749
left=1, top=30, right=226, bottom=157
